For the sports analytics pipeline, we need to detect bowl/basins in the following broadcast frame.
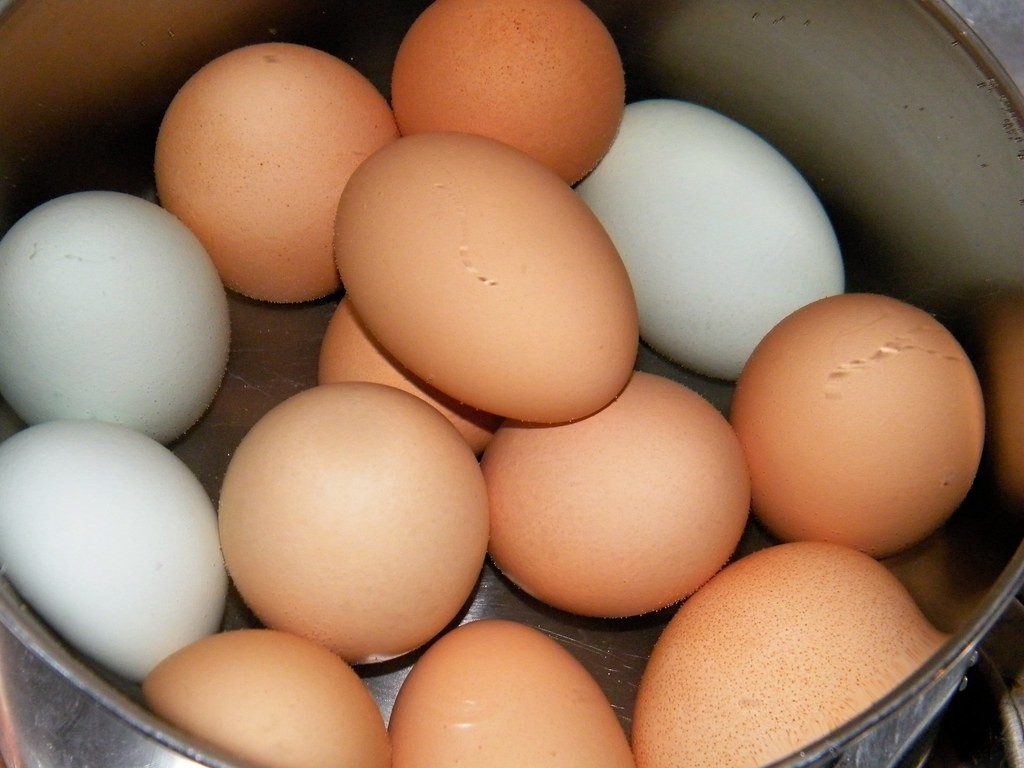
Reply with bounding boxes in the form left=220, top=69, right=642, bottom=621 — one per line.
left=0, top=0, right=1024, bottom=768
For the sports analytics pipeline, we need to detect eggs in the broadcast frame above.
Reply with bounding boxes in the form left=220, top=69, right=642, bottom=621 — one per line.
left=0, top=0, right=1024, bottom=768
